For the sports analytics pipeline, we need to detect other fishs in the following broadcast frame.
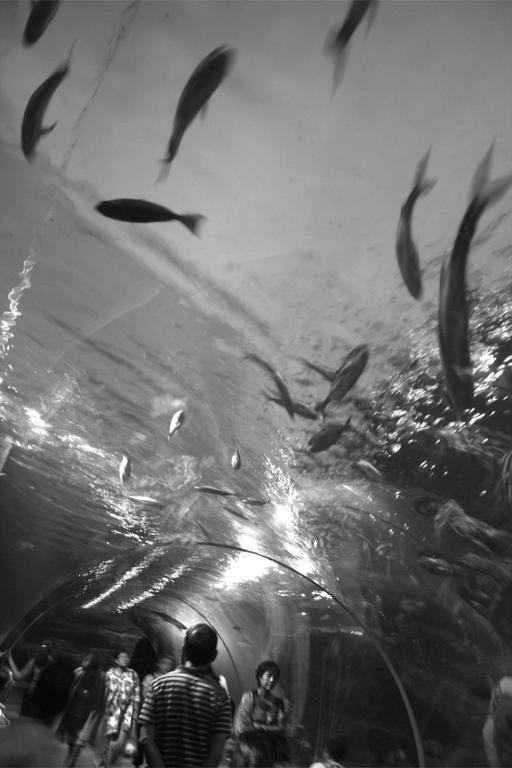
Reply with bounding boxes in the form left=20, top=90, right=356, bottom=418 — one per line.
left=119, top=454, right=164, bottom=511
left=231, top=450, right=243, bottom=471
left=243, top=344, right=378, bottom=482
left=188, top=482, right=269, bottom=523
left=167, top=401, right=187, bottom=442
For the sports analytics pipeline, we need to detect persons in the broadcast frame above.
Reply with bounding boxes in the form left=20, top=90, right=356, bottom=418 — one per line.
left=232, top=659, right=293, bottom=768
left=14, top=636, right=60, bottom=726
left=130, top=652, right=177, bottom=768
left=138, top=621, right=232, bottom=768
left=51, top=649, right=106, bottom=768
left=87, top=648, right=141, bottom=768
